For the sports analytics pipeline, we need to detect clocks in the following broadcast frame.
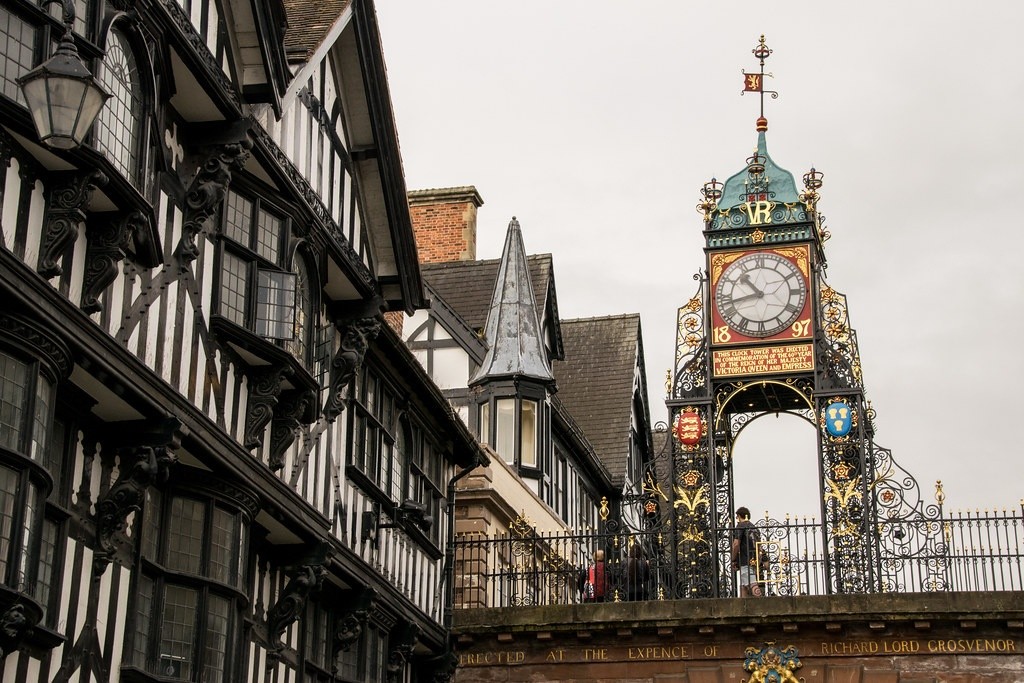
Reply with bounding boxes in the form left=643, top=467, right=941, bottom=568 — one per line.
left=715, top=254, right=806, bottom=338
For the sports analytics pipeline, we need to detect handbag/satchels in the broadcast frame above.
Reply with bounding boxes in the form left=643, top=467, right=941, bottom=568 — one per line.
left=583, top=581, right=594, bottom=599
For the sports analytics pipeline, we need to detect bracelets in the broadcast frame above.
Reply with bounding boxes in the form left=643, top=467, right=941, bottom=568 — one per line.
left=732, top=560, right=736, bottom=562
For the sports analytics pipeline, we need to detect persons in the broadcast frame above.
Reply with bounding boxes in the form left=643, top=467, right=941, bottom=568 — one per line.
left=583, top=550, right=609, bottom=603
left=731, top=507, right=769, bottom=598
left=621, top=544, right=649, bottom=601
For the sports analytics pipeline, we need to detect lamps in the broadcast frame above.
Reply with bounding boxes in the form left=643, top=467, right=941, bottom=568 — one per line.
left=16, top=0, right=115, bottom=154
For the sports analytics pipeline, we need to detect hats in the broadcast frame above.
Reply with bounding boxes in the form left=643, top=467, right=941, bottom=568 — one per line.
left=630, top=544, right=641, bottom=556
left=593, top=550, right=603, bottom=560
